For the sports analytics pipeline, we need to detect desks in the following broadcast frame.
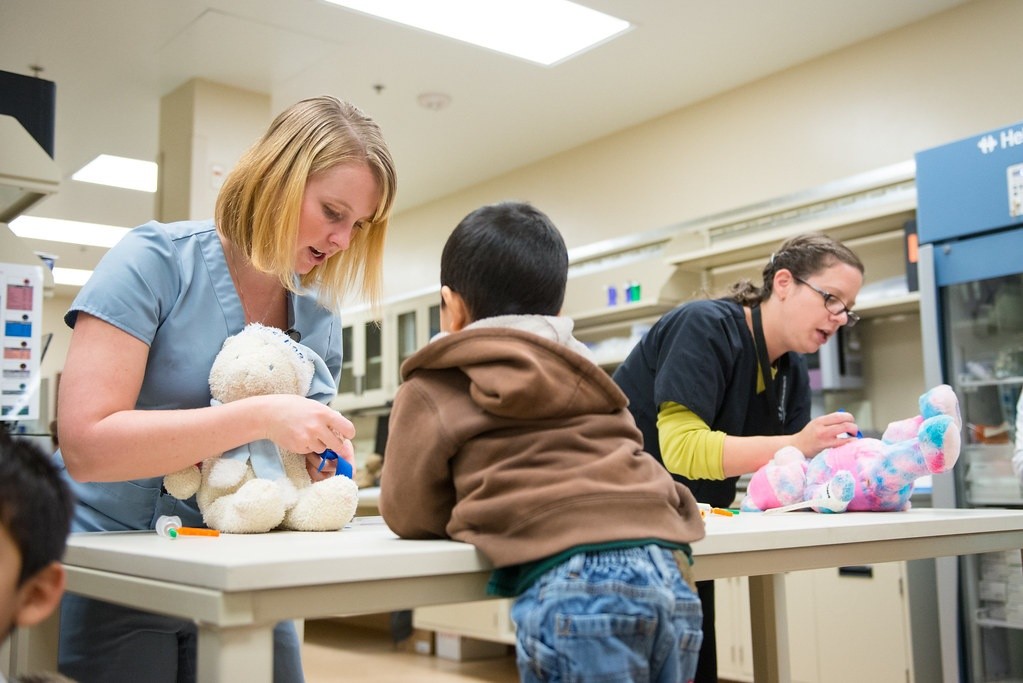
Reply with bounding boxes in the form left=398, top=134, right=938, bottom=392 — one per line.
left=13, top=507, right=1023, bottom=683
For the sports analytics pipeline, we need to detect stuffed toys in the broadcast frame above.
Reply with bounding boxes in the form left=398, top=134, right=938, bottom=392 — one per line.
left=741, top=384, right=963, bottom=514
left=163, top=323, right=358, bottom=531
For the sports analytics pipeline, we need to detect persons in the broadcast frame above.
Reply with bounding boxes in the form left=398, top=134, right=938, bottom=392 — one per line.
left=379, top=202, right=704, bottom=683
left=0, top=426, right=79, bottom=683
left=610, top=231, right=864, bottom=683
left=50, top=95, right=397, bottom=683
left=1014, top=387, right=1023, bottom=502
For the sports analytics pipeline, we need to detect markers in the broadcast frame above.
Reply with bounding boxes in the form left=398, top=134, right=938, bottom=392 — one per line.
left=834, top=406, right=864, bottom=440
left=697, top=506, right=740, bottom=519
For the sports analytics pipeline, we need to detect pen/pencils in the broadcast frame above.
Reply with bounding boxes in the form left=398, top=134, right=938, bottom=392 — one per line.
left=177, top=525, right=221, bottom=538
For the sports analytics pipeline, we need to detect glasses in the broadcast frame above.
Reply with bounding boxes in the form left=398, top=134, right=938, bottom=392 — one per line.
left=793, top=276, right=860, bottom=327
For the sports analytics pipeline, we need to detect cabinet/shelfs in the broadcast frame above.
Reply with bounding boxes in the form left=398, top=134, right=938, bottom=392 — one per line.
left=332, top=159, right=920, bottom=416
left=916, top=122, right=1022, bottom=683
